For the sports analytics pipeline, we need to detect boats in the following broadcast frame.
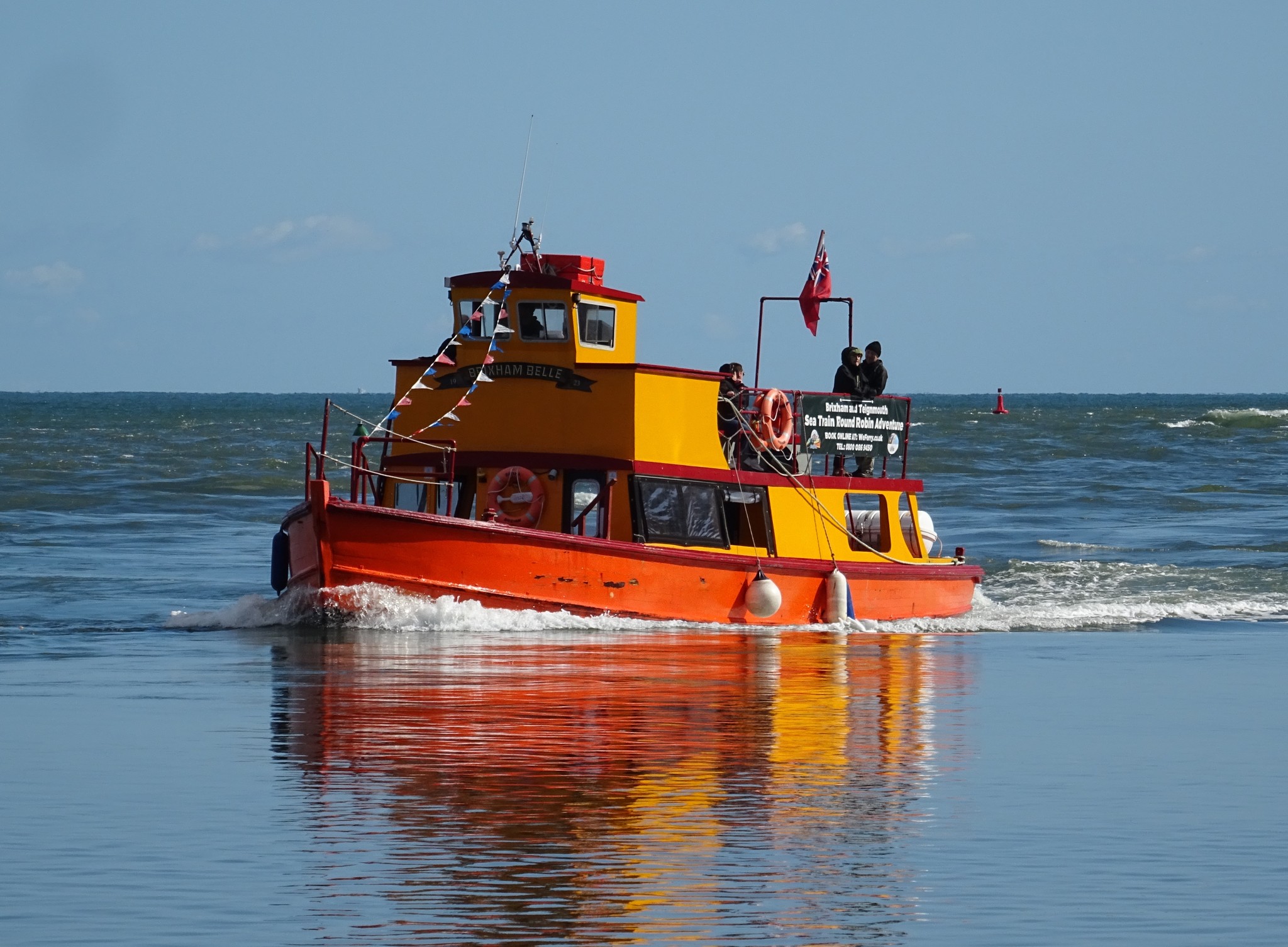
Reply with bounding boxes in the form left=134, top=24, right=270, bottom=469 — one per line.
left=271, top=112, right=987, bottom=626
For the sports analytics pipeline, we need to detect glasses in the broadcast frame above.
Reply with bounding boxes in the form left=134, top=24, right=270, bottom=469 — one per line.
left=741, top=372, right=745, bottom=376
left=730, top=364, right=734, bottom=371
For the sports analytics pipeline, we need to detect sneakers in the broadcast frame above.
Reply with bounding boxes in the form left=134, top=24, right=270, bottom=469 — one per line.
left=859, top=471, right=873, bottom=477
left=848, top=469, right=861, bottom=477
left=744, top=457, right=764, bottom=471
left=727, top=457, right=737, bottom=469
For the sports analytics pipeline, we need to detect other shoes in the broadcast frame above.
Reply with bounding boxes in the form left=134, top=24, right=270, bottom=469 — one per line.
left=772, top=459, right=793, bottom=472
left=833, top=468, right=852, bottom=477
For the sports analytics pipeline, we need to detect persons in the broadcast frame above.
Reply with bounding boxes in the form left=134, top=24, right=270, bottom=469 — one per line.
left=715, top=364, right=765, bottom=472
left=831, top=345, right=867, bottom=477
left=846, top=340, right=888, bottom=479
left=727, top=363, right=793, bottom=473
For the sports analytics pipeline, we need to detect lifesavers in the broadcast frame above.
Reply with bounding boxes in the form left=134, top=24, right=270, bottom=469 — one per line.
left=487, top=467, right=543, bottom=527
left=762, top=389, right=792, bottom=450
left=751, top=393, right=781, bottom=452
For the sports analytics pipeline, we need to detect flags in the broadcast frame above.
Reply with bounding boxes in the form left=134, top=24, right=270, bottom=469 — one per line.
left=798, top=230, right=832, bottom=337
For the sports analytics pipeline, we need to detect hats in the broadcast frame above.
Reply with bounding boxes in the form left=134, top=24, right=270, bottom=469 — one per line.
left=849, top=349, right=863, bottom=358
left=865, top=341, right=881, bottom=357
